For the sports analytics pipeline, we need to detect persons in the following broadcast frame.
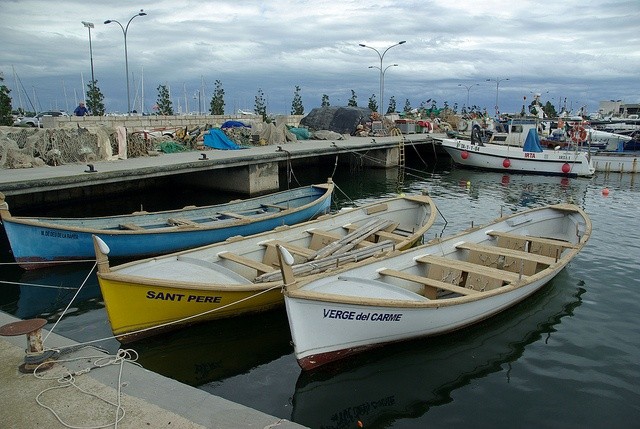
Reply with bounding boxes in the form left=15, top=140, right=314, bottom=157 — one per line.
left=74, top=102, right=90, bottom=116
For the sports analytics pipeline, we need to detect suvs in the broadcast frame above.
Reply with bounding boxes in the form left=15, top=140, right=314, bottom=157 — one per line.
left=20, top=111, right=69, bottom=127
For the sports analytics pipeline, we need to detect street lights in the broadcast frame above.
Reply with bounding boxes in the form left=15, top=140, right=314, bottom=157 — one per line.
left=103, top=13, right=148, bottom=115
left=458, top=84, right=479, bottom=112
left=81, top=21, right=95, bottom=87
left=357, top=38, right=406, bottom=115
left=366, top=65, right=401, bottom=116
left=486, top=79, right=509, bottom=122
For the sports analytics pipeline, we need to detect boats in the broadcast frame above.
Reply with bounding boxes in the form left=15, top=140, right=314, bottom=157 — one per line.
left=0, top=178, right=335, bottom=270
left=92, top=190, right=437, bottom=345
left=489, top=121, right=640, bottom=172
left=433, top=120, right=595, bottom=177
left=276, top=202, right=592, bottom=372
